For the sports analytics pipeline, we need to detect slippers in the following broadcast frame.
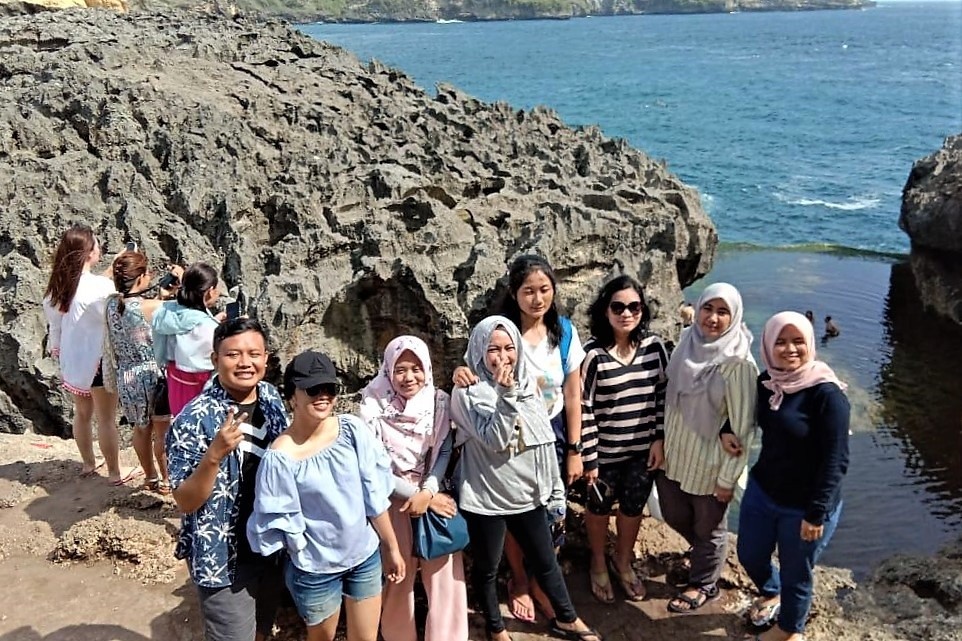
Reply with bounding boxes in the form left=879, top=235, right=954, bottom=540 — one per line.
left=591, top=560, right=615, bottom=602
left=508, top=578, right=535, bottom=622
left=107, top=466, right=144, bottom=485
left=752, top=594, right=781, bottom=625
left=549, top=616, right=604, bottom=641
left=612, top=558, right=646, bottom=601
left=747, top=624, right=803, bottom=641
left=81, top=455, right=107, bottom=476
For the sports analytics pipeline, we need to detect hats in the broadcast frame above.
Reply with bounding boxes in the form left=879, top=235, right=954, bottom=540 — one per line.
left=284, top=352, right=340, bottom=388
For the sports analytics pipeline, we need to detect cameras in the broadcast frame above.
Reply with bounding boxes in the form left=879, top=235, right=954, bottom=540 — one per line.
left=158, top=272, right=177, bottom=289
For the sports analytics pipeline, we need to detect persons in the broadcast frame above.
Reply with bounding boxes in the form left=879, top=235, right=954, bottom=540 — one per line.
left=823, top=315, right=840, bottom=338
left=245, top=349, right=407, bottom=641
left=805, top=309, right=814, bottom=325
left=164, top=315, right=292, bottom=641
left=719, top=311, right=853, bottom=641
left=680, top=301, right=696, bottom=328
left=453, top=255, right=587, bottom=621
left=449, top=314, right=606, bottom=641
left=42, top=227, right=144, bottom=486
left=357, top=333, right=471, bottom=641
left=152, top=262, right=248, bottom=422
left=575, top=272, right=672, bottom=601
left=652, top=281, right=762, bottom=613
left=105, top=251, right=187, bottom=495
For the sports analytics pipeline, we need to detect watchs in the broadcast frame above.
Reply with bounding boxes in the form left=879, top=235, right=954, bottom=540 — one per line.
left=568, top=440, right=584, bottom=453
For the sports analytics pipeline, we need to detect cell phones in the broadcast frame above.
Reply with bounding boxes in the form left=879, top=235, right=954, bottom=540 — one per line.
left=589, top=483, right=605, bottom=505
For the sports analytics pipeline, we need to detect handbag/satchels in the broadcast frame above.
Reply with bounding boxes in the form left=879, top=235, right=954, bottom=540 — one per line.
left=409, top=506, right=469, bottom=560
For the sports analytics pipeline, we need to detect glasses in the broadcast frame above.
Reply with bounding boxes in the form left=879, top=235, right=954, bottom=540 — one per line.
left=610, top=301, right=643, bottom=315
left=305, top=385, right=339, bottom=396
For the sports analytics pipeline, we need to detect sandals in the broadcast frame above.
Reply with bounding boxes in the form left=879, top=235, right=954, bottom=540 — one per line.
left=668, top=584, right=719, bottom=612
left=159, top=479, right=173, bottom=494
left=144, top=473, right=162, bottom=490
